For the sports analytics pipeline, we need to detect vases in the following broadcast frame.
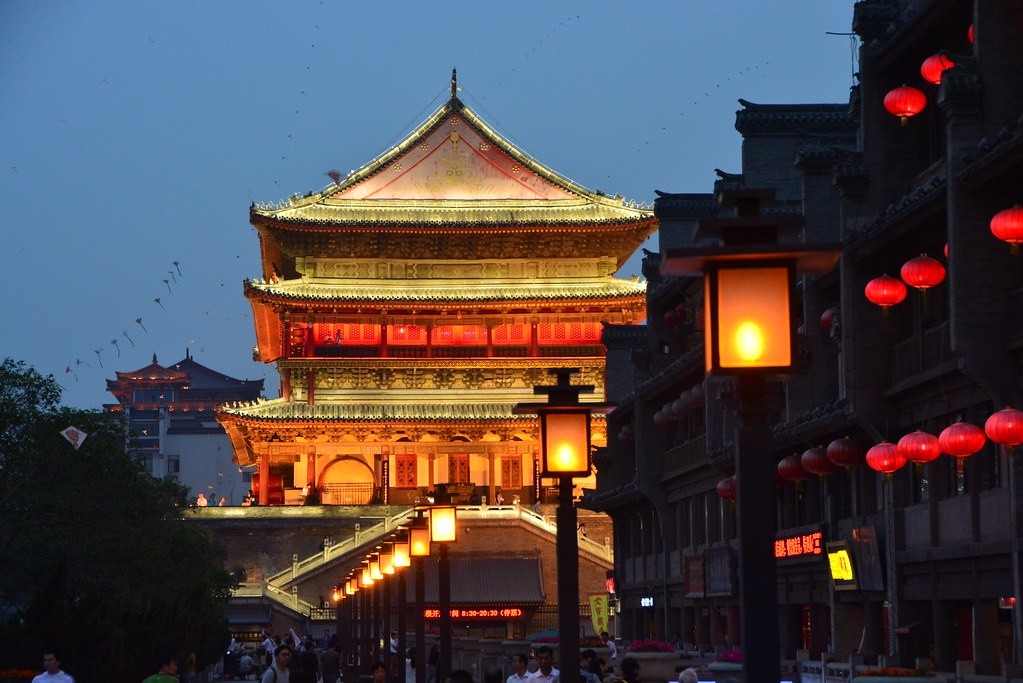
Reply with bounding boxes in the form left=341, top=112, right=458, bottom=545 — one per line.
left=625, top=649, right=680, bottom=681
left=850, top=675, right=948, bottom=683
left=579, top=647, right=615, bottom=670
left=405, top=633, right=560, bottom=664
left=707, top=661, right=745, bottom=682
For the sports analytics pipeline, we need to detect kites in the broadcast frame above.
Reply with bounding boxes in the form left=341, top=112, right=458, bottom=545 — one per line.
left=153, top=261, right=182, bottom=312
left=51, top=318, right=147, bottom=390
left=59, top=425, right=88, bottom=450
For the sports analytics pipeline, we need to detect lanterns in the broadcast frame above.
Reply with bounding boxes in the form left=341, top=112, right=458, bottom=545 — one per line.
left=665, top=303, right=687, bottom=337
left=653, top=373, right=738, bottom=429
left=777, top=435, right=865, bottom=485
left=865, top=243, right=948, bottom=307
left=821, top=309, right=834, bottom=331
left=1007, top=597, right=1016, bottom=606
left=968, top=24, right=974, bottom=43
left=865, top=406, right=1023, bottom=474
left=990, top=208, right=1023, bottom=256
left=884, top=85, right=926, bottom=126
left=717, top=477, right=738, bottom=502
left=617, top=424, right=633, bottom=441
left=921, top=54, right=955, bottom=85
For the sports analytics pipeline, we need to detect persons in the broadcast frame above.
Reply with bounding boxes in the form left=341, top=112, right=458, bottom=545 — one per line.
left=526, top=646, right=560, bottom=683
left=188, top=490, right=257, bottom=507
left=600, top=632, right=617, bottom=660
left=507, top=653, right=532, bottom=683
left=32, top=650, right=74, bottom=683
left=141, top=651, right=182, bottom=683
left=370, top=631, right=503, bottom=683
left=678, top=669, right=698, bottom=683
left=580, top=648, right=642, bottom=683
left=211, top=628, right=343, bottom=683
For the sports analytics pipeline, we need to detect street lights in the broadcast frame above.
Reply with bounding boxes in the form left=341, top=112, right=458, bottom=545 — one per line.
left=399, top=510, right=430, bottom=683
left=361, top=559, right=374, bottom=671
left=414, top=484, right=464, bottom=683
left=362, top=551, right=383, bottom=670
left=376, top=540, right=394, bottom=683
left=330, top=567, right=365, bottom=671
left=512, top=367, right=617, bottom=683
left=660, top=200, right=845, bottom=683
left=384, top=530, right=411, bottom=683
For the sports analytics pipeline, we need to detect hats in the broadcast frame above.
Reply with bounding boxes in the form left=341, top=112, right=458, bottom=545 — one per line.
left=301, top=635, right=308, bottom=640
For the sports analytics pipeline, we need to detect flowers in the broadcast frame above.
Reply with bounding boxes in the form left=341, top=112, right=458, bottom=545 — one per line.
left=858, top=666, right=936, bottom=678
left=579, top=640, right=608, bottom=649
left=715, top=646, right=745, bottom=666
left=406, top=628, right=559, bottom=642
left=631, top=640, right=678, bottom=655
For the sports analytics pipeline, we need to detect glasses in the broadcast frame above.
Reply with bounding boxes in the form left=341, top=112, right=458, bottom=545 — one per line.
left=278, top=652, right=291, bottom=658
